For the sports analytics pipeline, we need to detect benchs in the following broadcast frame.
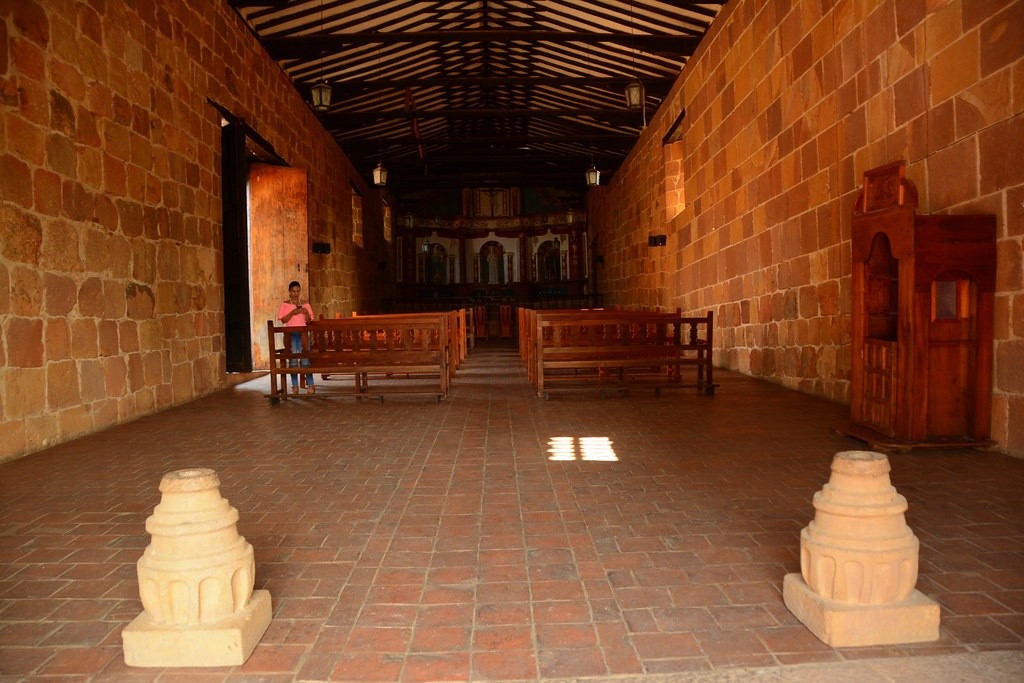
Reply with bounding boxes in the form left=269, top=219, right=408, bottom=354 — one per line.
left=517, top=305, right=720, bottom=400
left=266, top=308, right=475, bottom=402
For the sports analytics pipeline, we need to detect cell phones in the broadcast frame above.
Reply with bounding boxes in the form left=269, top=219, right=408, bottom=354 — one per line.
left=297, top=305, right=302, bottom=309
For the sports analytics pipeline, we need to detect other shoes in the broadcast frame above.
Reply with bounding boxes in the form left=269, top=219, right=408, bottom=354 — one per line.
left=307, top=386, right=315, bottom=393
left=291, top=387, right=299, bottom=393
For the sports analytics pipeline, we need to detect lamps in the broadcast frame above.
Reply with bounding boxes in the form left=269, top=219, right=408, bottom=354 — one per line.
left=585, top=43, right=600, bottom=186
left=372, top=41, right=389, bottom=185
left=421, top=238, right=431, bottom=255
left=311, top=1, right=332, bottom=111
left=552, top=237, right=561, bottom=254
left=625, top=76, right=648, bottom=136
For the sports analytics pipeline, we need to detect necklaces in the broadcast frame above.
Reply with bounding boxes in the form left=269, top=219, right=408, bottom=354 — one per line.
left=289, top=298, right=301, bottom=305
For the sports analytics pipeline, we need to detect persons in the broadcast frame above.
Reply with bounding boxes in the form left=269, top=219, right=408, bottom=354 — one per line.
left=278, top=281, right=314, bottom=399
